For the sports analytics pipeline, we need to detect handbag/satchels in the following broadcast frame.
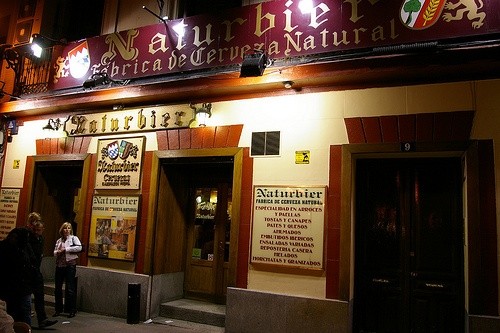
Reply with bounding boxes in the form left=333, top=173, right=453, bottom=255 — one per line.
left=56, top=244, right=83, bottom=265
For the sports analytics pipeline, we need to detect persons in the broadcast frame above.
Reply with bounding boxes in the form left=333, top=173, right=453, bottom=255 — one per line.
left=0, top=212, right=59, bottom=333
left=53, top=222, right=83, bottom=319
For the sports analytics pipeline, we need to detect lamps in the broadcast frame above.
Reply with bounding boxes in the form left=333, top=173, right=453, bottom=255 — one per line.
left=43, top=118, right=63, bottom=131
left=195, top=103, right=211, bottom=127
left=239, top=49, right=269, bottom=77
left=25, top=33, right=67, bottom=62
left=82, top=73, right=124, bottom=91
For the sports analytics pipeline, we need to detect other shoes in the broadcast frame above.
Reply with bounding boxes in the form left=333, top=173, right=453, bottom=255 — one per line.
left=70, top=312, right=75, bottom=317
left=53, top=312, right=60, bottom=316
left=39, top=319, right=58, bottom=328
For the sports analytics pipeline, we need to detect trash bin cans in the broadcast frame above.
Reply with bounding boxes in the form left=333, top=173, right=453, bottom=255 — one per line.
left=127, top=283, right=141, bottom=324
